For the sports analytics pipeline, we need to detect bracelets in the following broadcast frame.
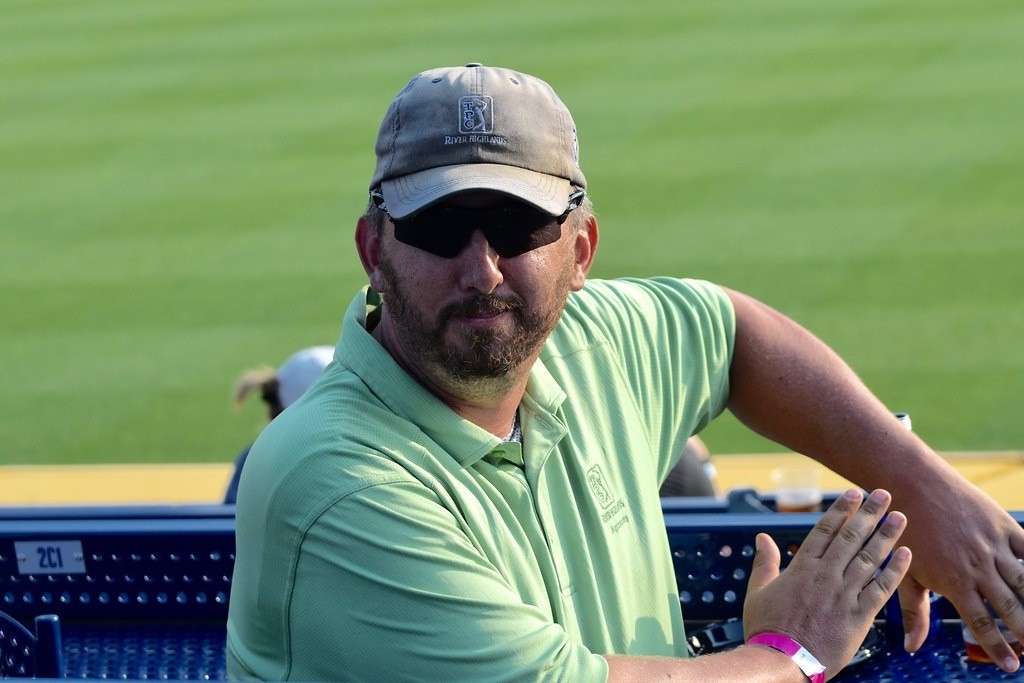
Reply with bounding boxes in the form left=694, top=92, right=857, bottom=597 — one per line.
left=746, top=632, right=826, bottom=683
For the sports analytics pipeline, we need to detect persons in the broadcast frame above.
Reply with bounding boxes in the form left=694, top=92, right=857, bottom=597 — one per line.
left=222, top=346, right=335, bottom=504
left=226, top=65, right=1024, bottom=683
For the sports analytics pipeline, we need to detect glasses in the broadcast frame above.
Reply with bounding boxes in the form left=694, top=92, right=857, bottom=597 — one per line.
left=370, top=184, right=584, bottom=259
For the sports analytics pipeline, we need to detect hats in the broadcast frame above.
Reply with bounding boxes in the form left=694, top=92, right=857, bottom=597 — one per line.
left=369, top=63, right=587, bottom=219
left=276, top=346, right=335, bottom=409
